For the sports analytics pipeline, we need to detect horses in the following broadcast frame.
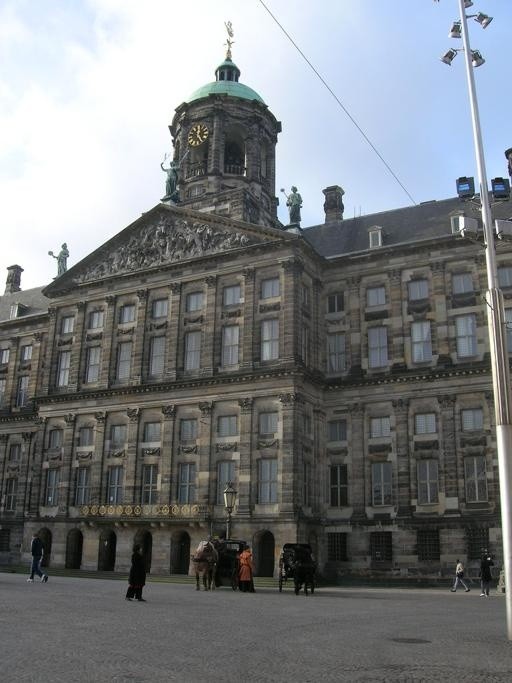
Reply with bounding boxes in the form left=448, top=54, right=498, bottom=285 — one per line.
left=293, top=546, right=314, bottom=596
left=191, top=541, right=219, bottom=591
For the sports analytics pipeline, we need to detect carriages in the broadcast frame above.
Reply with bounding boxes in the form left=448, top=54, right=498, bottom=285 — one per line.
left=189, top=537, right=250, bottom=593
left=276, top=541, right=319, bottom=596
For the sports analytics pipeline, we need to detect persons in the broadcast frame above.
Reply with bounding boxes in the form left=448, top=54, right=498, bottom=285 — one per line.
left=57, top=243, right=69, bottom=276
left=238, top=545, right=255, bottom=593
left=26, top=533, right=46, bottom=583
left=451, top=559, right=470, bottom=592
left=479, top=554, right=494, bottom=596
left=125, top=543, right=147, bottom=602
left=286, top=186, right=303, bottom=227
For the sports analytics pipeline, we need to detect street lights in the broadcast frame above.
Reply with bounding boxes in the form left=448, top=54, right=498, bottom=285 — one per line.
left=448, top=0, right=512, bottom=663
left=223, top=480, right=239, bottom=542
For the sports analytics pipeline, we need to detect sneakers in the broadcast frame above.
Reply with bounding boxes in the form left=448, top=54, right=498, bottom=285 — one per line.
left=41, top=574, right=46, bottom=583
left=124, top=597, right=147, bottom=602
left=27, top=579, right=35, bottom=583
left=451, top=589, right=456, bottom=592
left=479, top=593, right=489, bottom=598
left=464, top=589, right=470, bottom=592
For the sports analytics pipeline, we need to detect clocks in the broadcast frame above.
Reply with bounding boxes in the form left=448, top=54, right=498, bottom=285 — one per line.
left=185, top=122, right=210, bottom=145
